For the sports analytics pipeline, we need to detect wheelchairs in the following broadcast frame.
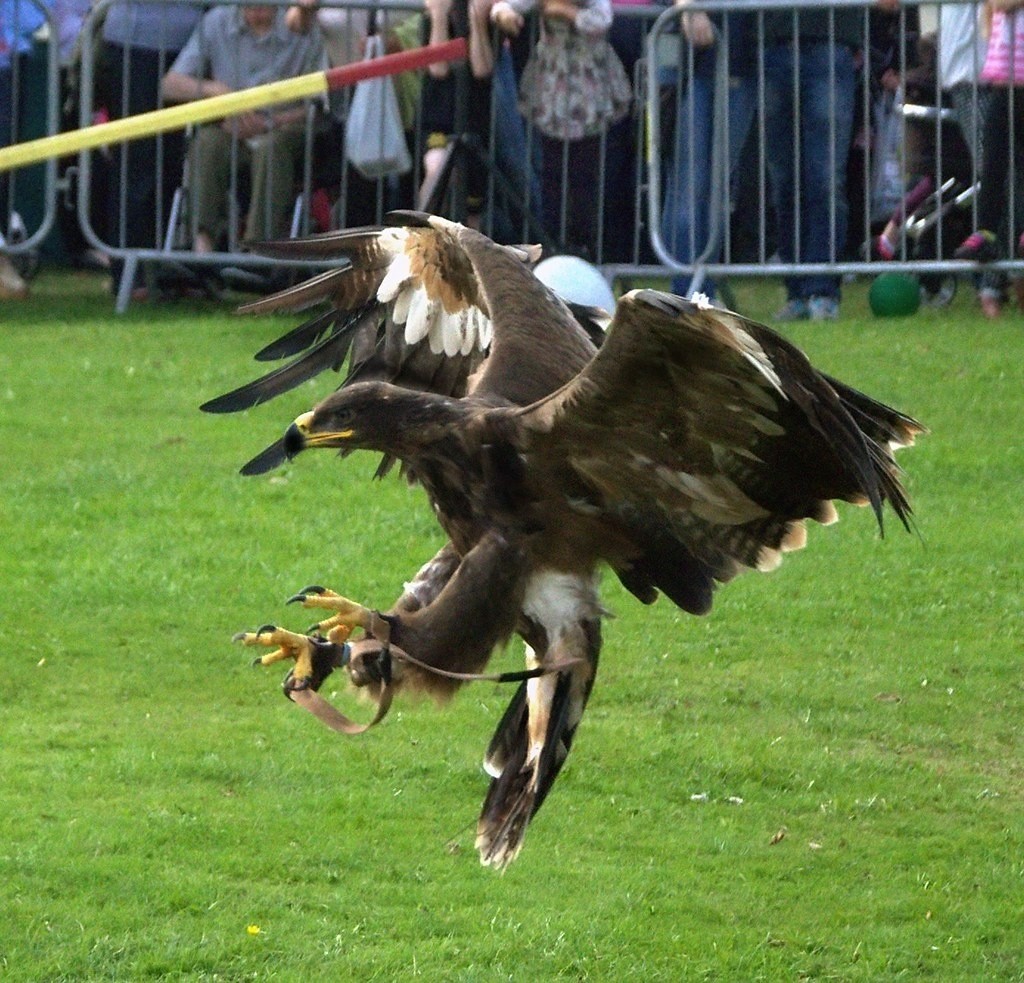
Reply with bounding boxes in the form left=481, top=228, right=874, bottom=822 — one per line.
left=153, top=116, right=306, bottom=305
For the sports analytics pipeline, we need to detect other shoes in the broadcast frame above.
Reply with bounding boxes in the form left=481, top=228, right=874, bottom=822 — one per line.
left=781, top=295, right=841, bottom=321
left=171, top=254, right=272, bottom=289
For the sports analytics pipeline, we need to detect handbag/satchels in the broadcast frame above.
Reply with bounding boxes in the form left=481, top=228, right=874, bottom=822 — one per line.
left=345, top=37, right=413, bottom=178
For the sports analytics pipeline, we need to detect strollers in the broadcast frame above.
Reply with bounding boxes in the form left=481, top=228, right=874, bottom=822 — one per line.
left=878, top=102, right=982, bottom=310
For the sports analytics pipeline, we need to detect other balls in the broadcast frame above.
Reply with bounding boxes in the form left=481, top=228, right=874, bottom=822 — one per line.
left=868, top=270, right=921, bottom=315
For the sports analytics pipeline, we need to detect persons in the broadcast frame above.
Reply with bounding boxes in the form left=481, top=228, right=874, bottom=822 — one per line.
left=0, top=0, right=1024, bottom=320
